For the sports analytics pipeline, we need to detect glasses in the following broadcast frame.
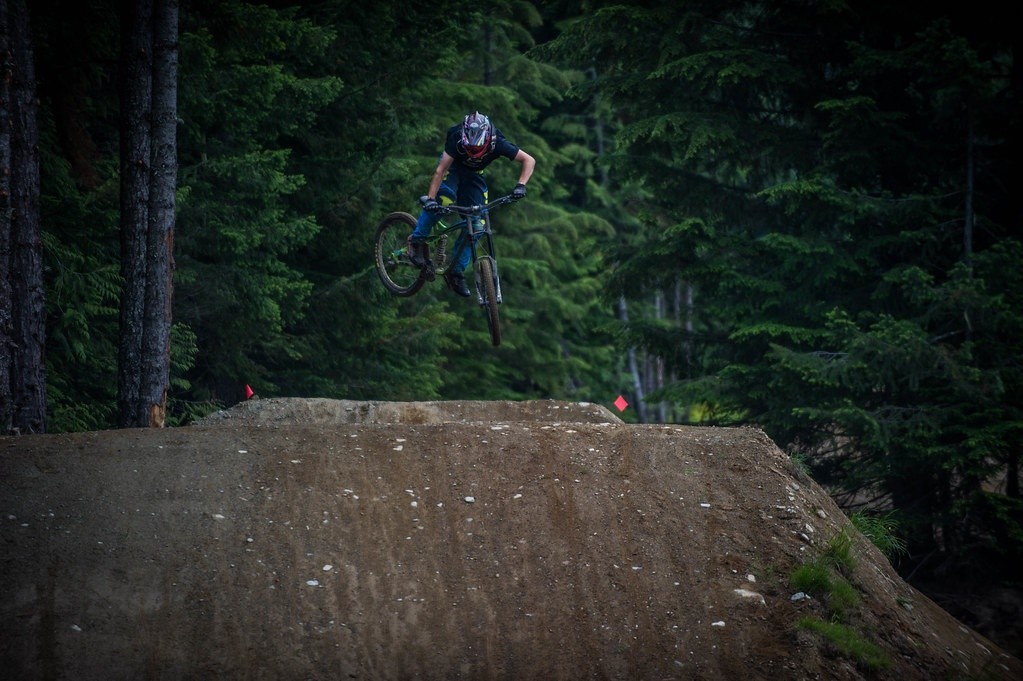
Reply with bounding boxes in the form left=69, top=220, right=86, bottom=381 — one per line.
left=465, top=140, right=488, bottom=151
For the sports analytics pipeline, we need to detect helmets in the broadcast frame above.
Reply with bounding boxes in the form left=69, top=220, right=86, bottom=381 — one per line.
left=461, top=111, right=496, bottom=163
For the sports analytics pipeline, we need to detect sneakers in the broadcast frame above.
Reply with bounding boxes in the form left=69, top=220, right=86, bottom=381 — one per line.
left=407, top=234, right=424, bottom=267
left=444, top=271, right=471, bottom=297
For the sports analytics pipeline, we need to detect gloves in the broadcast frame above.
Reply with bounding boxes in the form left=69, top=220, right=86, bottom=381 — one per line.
left=424, top=198, right=439, bottom=211
left=513, top=184, right=527, bottom=198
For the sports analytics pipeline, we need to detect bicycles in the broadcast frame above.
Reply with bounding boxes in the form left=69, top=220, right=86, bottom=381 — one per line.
left=375, top=194, right=529, bottom=347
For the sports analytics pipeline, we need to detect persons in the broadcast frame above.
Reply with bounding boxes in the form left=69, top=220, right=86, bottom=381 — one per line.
left=407, top=111, right=535, bottom=297
